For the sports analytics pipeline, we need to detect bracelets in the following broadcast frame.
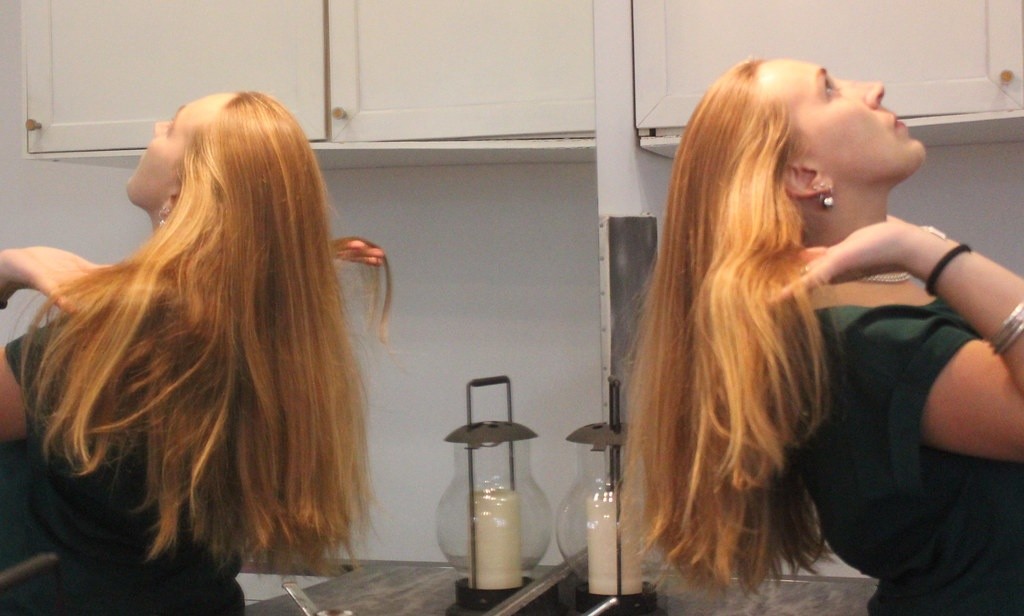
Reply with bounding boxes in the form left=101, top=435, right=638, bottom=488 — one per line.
left=989, top=300, right=1024, bottom=355
left=926, top=243, right=970, bottom=296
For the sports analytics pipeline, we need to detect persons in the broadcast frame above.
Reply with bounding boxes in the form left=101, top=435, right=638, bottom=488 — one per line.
left=620, top=57, right=1024, bottom=616
left=0, top=88, right=395, bottom=616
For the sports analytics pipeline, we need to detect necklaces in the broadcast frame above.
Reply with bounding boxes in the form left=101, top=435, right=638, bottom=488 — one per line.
left=861, top=272, right=912, bottom=282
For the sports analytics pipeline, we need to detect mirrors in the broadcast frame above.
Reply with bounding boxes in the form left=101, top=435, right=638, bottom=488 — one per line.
left=0, top=0, right=611, bottom=615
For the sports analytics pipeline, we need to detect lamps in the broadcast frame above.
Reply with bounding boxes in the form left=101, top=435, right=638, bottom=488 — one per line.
left=553, top=381, right=659, bottom=615
left=433, top=378, right=554, bottom=611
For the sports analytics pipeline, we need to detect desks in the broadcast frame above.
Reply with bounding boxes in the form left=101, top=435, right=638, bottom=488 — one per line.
left=247, top=560, right=558, bottom=613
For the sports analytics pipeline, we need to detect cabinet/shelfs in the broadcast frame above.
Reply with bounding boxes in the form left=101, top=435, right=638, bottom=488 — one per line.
left=18, top=0, right=595, bottom=171
left=628, top=1, right=1024, bottom=158
